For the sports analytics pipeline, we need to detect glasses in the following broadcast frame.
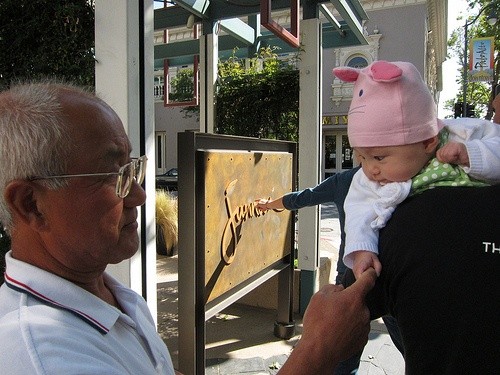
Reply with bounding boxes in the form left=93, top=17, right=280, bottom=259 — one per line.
left=493, top=84, right=500, bottom=96
left=29, top=155, right=147, bottom=199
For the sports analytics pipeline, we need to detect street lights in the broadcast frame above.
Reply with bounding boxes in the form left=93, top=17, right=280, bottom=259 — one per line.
left=463, top=2, right=498, bottom=118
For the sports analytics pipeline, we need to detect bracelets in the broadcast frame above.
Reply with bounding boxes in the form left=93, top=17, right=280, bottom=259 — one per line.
left=266, top=201, right=271, bottom=210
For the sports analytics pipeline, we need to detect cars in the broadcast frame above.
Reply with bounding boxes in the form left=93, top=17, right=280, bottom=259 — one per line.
left=156, top=168, right=177, bottom=191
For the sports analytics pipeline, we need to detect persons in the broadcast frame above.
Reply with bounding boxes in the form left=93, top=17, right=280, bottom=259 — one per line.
left=332, top=60, right=500, bottom=280
left=254, top=150, right=363, bottom=285
left=375, top=54, right=500, bottom=375
left=1, top=78, right=377, bottom=375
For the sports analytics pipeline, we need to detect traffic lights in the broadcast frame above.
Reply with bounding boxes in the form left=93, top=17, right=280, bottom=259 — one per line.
left=465, top=103, right=475, bottom=117
left=455, top=103, right=462, bottom=118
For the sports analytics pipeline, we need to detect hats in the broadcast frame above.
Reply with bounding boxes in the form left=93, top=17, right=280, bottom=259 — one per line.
left=332, top=61, right=439, bottom=147
